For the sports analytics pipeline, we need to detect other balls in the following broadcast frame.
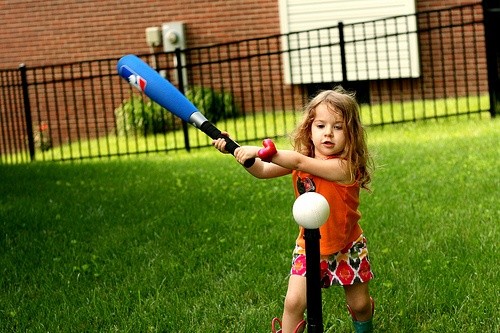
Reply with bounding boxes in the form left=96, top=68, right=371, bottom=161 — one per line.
left=292, top=191, right=331, bottom=230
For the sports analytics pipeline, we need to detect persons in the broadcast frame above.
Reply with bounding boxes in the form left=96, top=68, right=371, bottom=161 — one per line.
left=212, top=86, right=377, bottom=332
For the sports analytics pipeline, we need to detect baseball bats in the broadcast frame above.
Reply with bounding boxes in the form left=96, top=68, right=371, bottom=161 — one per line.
left=116, top=54, right=255, bottom=168
